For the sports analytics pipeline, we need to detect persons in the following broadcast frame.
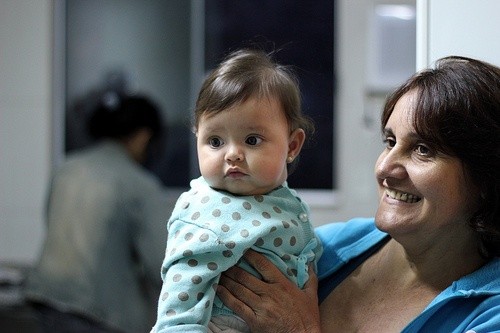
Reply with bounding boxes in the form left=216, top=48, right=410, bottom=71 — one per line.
left=150, top=49, right=325, bottom=333
left=18, top=89, right=170, bottom=333
left=208, top=55, right=500, bottom=333
